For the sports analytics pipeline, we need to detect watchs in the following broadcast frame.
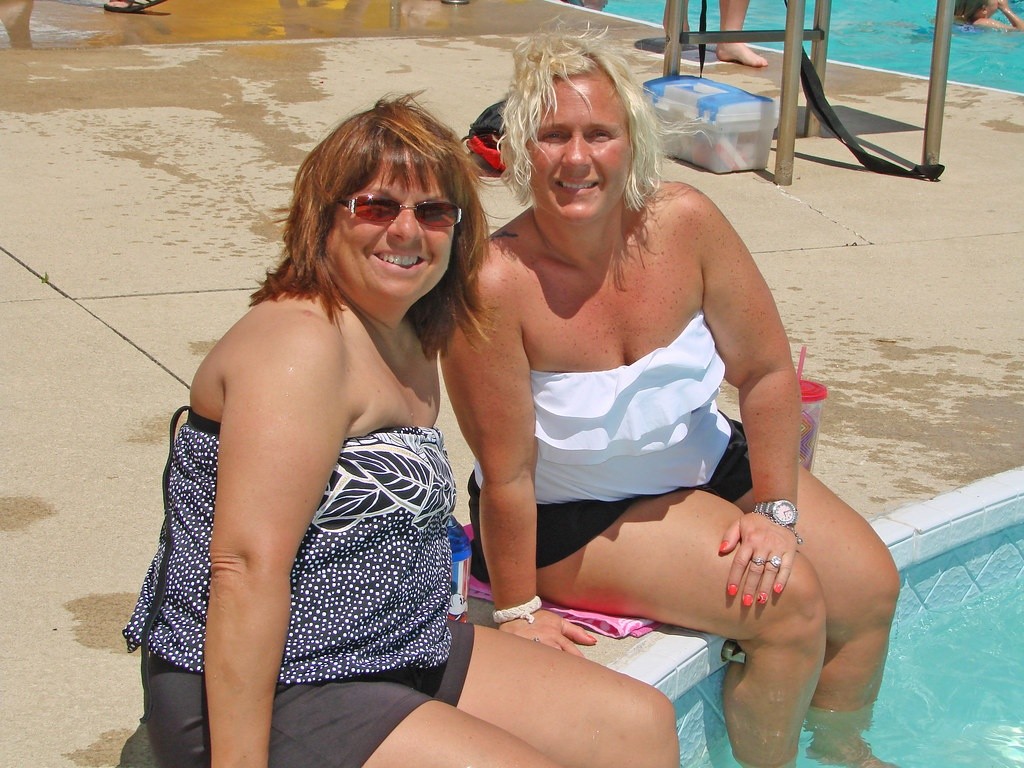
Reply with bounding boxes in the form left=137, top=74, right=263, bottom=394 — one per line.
left=753, top=501, right=798, bottom=525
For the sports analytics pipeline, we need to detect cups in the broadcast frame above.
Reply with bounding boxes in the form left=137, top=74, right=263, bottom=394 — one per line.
left=799, top=380, right=828, bottom=471
left=447, top=514, right=472, bottom=624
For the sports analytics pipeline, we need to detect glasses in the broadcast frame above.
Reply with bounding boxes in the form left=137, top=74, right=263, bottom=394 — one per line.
left=336, top=195, right=462, bottom=227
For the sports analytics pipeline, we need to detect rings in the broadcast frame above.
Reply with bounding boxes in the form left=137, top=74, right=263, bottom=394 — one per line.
left=766, top=556, right=782, bottom=568
left=750, top=557, right=766, bottom=567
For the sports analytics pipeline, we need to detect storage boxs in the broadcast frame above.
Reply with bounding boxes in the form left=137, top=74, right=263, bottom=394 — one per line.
left=643, top=75, right=779, bottom=174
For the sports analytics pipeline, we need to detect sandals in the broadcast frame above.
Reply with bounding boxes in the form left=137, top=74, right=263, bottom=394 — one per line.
left=103, top=0, right=168, bottom=13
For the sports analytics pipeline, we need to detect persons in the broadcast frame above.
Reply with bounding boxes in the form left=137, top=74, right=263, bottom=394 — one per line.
left=662, top=0, right=768, bottom=68
left=104, top=0, right=171, bottom=13
left=119, top=85, right=687, bottom=766
left=436, top=15, right=899, bottom=765
left=953, top=0, right=1024, bottom=32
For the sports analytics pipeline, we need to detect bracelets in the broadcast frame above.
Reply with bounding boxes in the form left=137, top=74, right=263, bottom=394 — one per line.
left=493, top=594, right=543, bottom=625
left=757, top=510, right=803, bottom=544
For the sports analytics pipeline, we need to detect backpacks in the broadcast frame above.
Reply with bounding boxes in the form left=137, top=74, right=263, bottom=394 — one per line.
left=461, top=100, right=506, bottom=172
